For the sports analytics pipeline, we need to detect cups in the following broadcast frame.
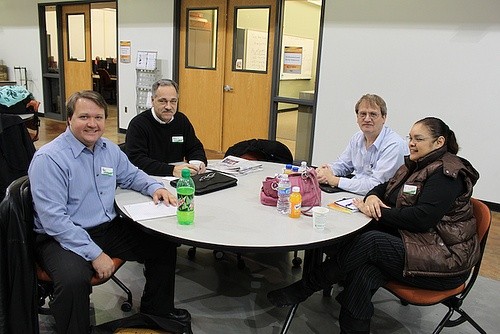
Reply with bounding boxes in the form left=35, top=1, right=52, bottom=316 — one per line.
left=312, top=206, right=330, bottom=230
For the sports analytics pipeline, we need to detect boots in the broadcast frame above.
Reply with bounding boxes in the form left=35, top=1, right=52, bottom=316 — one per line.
left=267, top=255, right=344, bottom=308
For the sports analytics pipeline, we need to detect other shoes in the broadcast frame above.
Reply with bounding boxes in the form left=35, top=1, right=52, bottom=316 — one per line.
left=159, top=309, right=191, bottom=323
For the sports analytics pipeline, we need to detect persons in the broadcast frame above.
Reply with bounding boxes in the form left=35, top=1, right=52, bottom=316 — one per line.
left=28, top=90, right=192, bottom=334
left=124, top=78, right=208, bottom=178
left=315, top=92, right=405, bottom=196
left=267, top=118, right=480, bottom=334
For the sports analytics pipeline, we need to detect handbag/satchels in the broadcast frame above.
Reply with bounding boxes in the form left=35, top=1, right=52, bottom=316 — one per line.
left=261, top=169, right=321, bottom=207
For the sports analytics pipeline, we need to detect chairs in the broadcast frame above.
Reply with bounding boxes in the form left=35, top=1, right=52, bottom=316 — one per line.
left=97, top=68, right=117, bottom=104
left=0, top=115, right=491, bottom=334
left=0, top=86, right=39, bottom=142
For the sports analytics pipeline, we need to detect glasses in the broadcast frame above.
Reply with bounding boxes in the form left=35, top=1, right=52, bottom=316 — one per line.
left=357, top=111, right=382, bottom=119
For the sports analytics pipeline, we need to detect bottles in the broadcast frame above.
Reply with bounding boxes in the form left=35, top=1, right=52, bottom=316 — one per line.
left=283, top=161, right=309, bottom=175
left=176, top=168, right=195, bottom=225
left=289, top=187, right=302, bottom=219
left=277, top=174, right=291, bottom=215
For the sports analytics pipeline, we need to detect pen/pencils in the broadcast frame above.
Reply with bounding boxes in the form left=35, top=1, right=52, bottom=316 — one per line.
left=157, top=198, right=163, bottom=203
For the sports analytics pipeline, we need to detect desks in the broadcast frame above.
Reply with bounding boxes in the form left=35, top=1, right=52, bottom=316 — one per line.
left=113, top=160, right=374, bottom=334
left=93, top=75, right=117, bottom=95
